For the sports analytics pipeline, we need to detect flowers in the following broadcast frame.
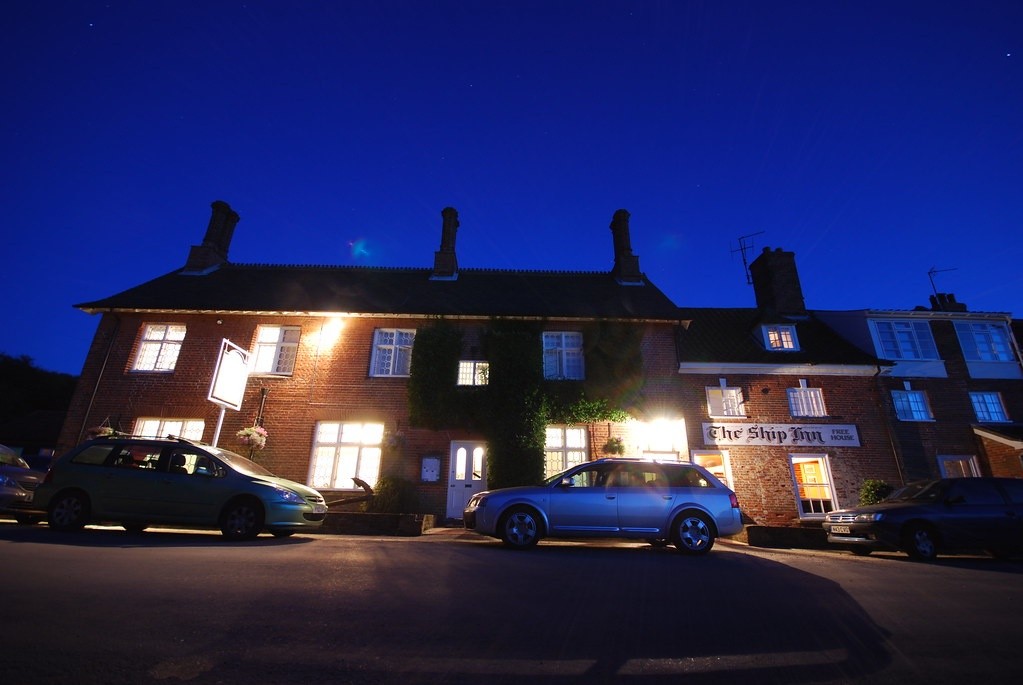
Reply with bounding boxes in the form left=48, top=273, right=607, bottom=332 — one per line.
left=235, top=425, right=268, bottom=451
left=601, top=436, right=625, bottom=456
left=84, top=426, right=114, bottom=440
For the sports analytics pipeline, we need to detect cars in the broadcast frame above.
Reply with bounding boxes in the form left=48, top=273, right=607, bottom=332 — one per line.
left=0, top=445, right=49, bottom=527
left=464, top=457, right=746, bottom=555
left=820, top=476, right=1023, bottom=567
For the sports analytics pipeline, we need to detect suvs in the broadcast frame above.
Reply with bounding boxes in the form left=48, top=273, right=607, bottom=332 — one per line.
left=35, top=433, right=328, bottom=544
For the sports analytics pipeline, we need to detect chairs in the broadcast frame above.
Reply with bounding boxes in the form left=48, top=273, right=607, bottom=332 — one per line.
left=191, top=457, right=213, bottom=477
left=170, top=454, right=188, bottom=474
left=117, top=455, right=135, bottom=465
